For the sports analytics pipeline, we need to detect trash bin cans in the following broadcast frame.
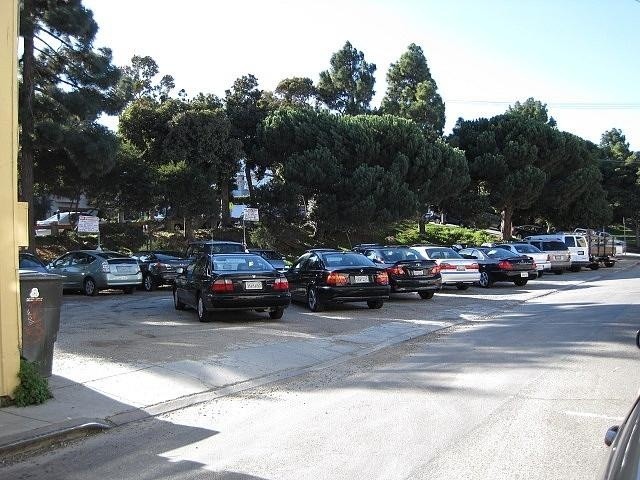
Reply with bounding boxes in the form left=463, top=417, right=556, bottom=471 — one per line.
left=20, top=274, right=67, bottom=378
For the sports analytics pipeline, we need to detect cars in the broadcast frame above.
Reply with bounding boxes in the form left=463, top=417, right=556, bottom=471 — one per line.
left=594, top=329, right=640, bottom=480
left=45, top=250, right=143, bottom=296
left=172, top=254, right=292, bottom=322
left=18, top=252, right=50, bottom=274
left=346, top=230, right=624, bottom=300
left=36, top=212, right=114, bottom=238
left=282, top=249, right=391, bottom=312
left=120, top=251, right=190, bottom=291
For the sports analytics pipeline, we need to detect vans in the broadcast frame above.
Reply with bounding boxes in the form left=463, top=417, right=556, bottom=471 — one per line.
left=142, top=205, right=221, bottom=234
left=182, top=240, right=247, bottom=275
left=246, top=249, right=288, bottom=276
